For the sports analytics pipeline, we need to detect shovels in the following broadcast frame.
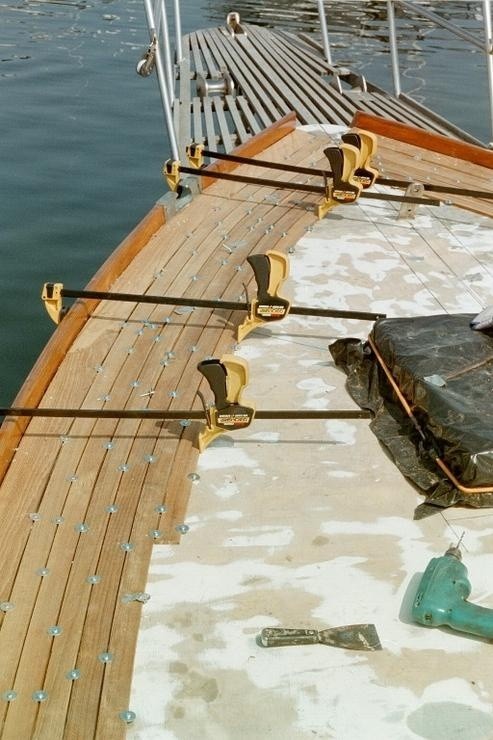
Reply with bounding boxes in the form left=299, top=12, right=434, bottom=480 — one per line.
left=258, top=623, right=384, bottom=652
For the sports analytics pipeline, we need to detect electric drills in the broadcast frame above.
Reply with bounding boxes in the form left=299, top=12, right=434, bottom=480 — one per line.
left=410, top=531, right=493, bottom=646
